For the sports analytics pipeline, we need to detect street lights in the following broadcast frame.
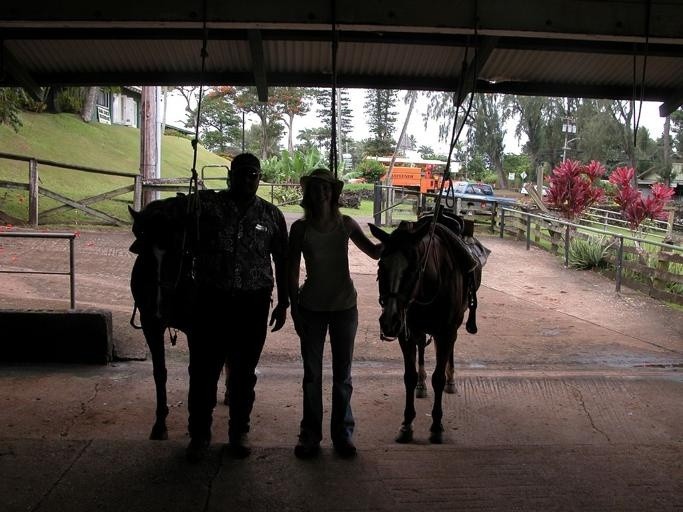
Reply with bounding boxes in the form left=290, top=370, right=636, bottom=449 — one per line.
left=561, top=116, right=583, bottom=163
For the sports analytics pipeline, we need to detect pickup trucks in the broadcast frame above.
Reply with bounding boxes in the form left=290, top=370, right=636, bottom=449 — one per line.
left=437, top=182, right=515, bottom=210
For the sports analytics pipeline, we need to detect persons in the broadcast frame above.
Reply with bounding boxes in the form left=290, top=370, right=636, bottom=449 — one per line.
left=167, top=153, right=288, bottom=466
left=291, top=168, right=415, bottom=462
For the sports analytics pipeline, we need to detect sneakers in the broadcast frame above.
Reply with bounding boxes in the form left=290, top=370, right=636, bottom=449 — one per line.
left=333, top=439, right=356, bottom=458
left=228, top=430, right=251, bottom=456
left=186, top=440, right=211, bottom=462
left=294, top=442, right=320, bottom=460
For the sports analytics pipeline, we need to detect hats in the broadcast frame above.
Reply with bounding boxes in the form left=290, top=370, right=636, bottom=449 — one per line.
left=231, top=153, right=260, bottom=170
left=300, top=168, right=344, bottom=193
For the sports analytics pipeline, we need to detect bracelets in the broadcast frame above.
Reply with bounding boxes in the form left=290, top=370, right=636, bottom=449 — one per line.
left=279, top=300, right=290, bottom=308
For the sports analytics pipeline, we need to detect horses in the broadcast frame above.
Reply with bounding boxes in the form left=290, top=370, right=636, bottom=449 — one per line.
left=127, top=197, right=231, bottom=442
left=368, top=221, right=483, bottom=444
